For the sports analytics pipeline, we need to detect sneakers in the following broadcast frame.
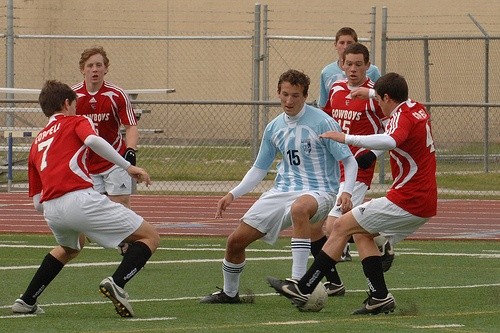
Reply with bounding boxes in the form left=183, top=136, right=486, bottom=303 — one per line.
left=99, top=276, right=134, bottom=317
left=352, top=291, right=396, bottom=315
left=339, top=242, right=352, bottom=261
left=200, top=286, right=241, bottom=304
left=323, top=280, right=345, bottom=297
left=12, top=294, right=46, bottom=315
left=378, top=239, right=394, bottom=272
left=266, top=276, right=312, bottom=307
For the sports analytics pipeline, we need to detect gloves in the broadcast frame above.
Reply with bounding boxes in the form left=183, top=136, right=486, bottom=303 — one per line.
left=356, top=150, right=377, bottom=169
left=123, top=147, right=138, bottom=166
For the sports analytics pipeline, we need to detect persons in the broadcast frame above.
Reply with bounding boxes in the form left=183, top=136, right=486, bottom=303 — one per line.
left=12, top=79, right=160, bottom=318
left=318, top=27, right=381, bottom=262
left=321, top=44, right=396, bottom=296
left=200, top=70, right=358, bottom=303
left=265, top=72, right=437, bottom=316
left=69, top=47, right=139, bottom=254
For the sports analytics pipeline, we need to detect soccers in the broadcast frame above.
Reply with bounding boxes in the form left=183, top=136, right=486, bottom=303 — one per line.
left=299, top=281, right=329, bottom=313
